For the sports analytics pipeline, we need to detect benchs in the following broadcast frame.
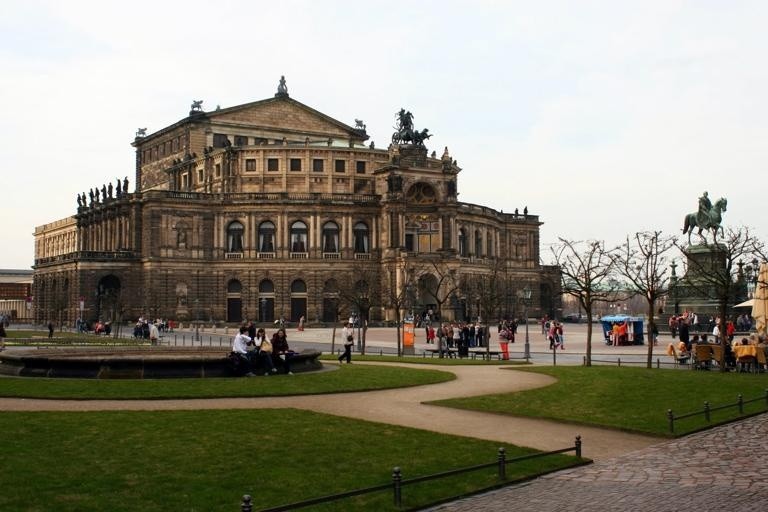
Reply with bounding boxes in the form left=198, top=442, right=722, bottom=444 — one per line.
left=426, top=349, right=458, bottom=358
left=469, top=351, right=507, bottom=360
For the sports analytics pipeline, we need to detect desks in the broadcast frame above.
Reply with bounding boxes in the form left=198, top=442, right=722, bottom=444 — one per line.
left=735, top=346, right=766, bottom=374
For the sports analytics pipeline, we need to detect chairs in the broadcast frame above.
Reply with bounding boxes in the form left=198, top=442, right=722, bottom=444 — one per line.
left=669, top=341, right=738, bottom=372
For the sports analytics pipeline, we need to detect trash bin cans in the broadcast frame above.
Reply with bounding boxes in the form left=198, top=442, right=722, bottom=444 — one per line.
left=567, top=315, right=577, bottom=323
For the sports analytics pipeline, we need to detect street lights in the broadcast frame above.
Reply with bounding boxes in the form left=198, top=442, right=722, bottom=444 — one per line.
left=193, top=298, right=201, bottom=342
left=261, top=297, right=268, bottom=329
left=752, top=258, right=759, bottom=291
left=521, top=282, right=534, bottom=359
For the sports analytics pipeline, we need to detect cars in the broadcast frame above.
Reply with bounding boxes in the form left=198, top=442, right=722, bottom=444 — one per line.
left=565, top=312, right=648, bottom=324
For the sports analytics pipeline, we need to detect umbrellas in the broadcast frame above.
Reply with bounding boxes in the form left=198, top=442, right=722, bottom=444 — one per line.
left=751, top=261, right=768, bottom=335
left=732, top=299, right=756, bottom=308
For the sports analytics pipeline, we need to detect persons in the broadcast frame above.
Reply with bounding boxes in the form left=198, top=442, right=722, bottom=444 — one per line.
left=134, top=316, right=176, bottom=342
left=298, top=315, right=305, bottom=331
left=76, top=317, right=112, bottom=335
left=0, top=312, right=10, bottom=338
left=611, top=312, right=768, bottom=373
left=515, top=209, right=518, bottom=218
left=541, top=314, right=565, bottom=350
left=396, top=108, right=414, bottom=132
left=348, top=315, right=359, bottom=328
left=524, top=207, right=528, bottom=217
left=77, top=177, right=128, bottom=206
left=699, top=192, right=712, bottom=224
left=278, top=76, right=287, bottom=93
left=415, top=313, right=519, bottom=358
left=48, top=322, right=54, bottom=336
left=232, top=321, right=295, bottom=376
left=338, top=322, right=354, bottom=364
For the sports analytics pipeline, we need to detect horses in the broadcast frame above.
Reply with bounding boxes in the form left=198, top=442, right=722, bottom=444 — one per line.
left=683, top=198, right=727, bottom=246
